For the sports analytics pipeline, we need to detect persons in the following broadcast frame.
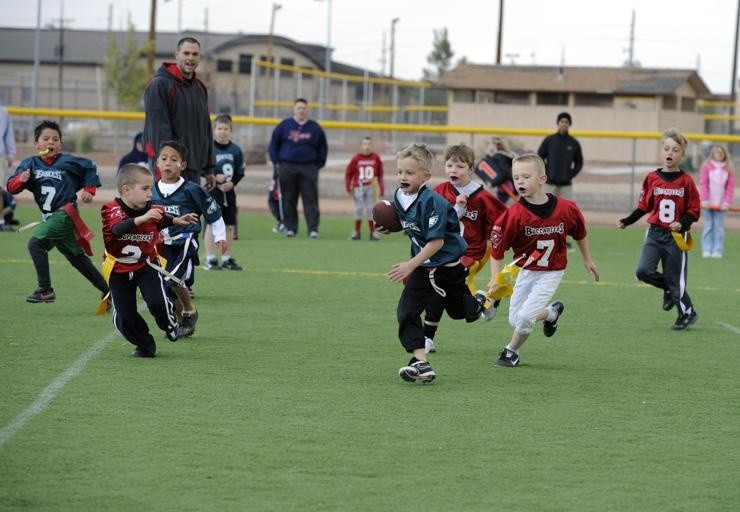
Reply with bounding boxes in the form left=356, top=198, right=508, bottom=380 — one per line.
left=373, top=142, right=488, bottom=385
left=1, top=114, right=20, bottom=231
left=617, top=128, right=701, bottom=330
left=699, top=142, right=735, bottom=259
left=101, top=164, right=198, bottom=357
left=345, top=138, right=385, bottom=241
left=482, top=136, right=518, bottom=204
left=141, top=37, right=216, bottom=298
left=537, top=111, right=583, bottom=251
left=150, top=143, right=227, bottom=338
left=118, top=114, right=244, bottom=271
left=421, top=143, right=509, bottom=353
left=7, top=120, right=111, bottom=309
left=486, top=152, right=600, bottom=368
left=268, top=98, right=328, bottom=238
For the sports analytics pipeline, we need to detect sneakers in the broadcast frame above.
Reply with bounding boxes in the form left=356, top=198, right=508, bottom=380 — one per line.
left=663, top=289, right=674, bottom=311
left=130, top=346, right=155, bottom=357
left=672, top=305, right=697, bottom=329
left=493, top=348, right=519, bottom=366
left=351, top=231, right=380, bottom=239
left=203, top=256, right=242, bottom=270
left=466, top=290, right=501, bottom=322
left=166, top=288, right=197, bottom=341
left=543, top=302, right=563, bottom=337
left=400, top=339, right=438, bottom=385
left=26, top=287, right=56, bottom=302
left=271, top=222, right=318, bottom=237
left=102, top=293, right=113, bottom=312
left=702, top=252, right=722, bottom=259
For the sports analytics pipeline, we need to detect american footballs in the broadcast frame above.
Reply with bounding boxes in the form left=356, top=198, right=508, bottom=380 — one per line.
left=374, top=200, right=402, bottom=233
